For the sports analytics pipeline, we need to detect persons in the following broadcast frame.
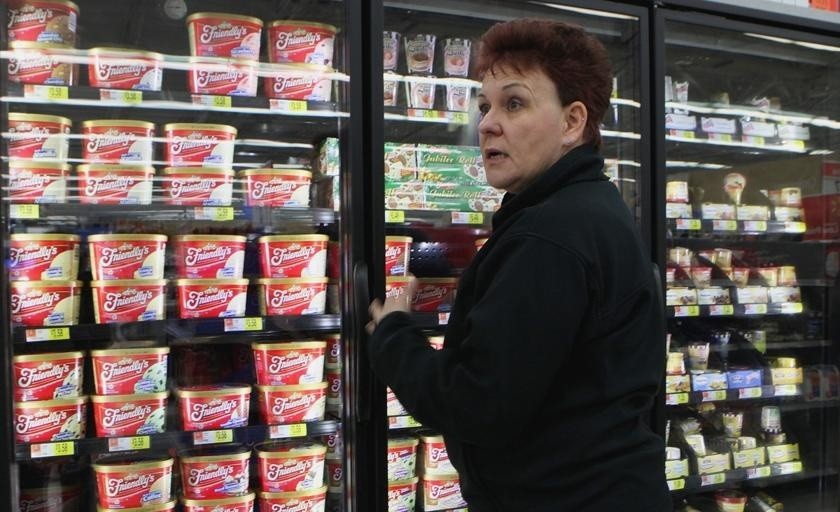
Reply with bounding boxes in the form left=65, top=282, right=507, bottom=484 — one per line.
left=362, top=14, right=686, bottom=511
